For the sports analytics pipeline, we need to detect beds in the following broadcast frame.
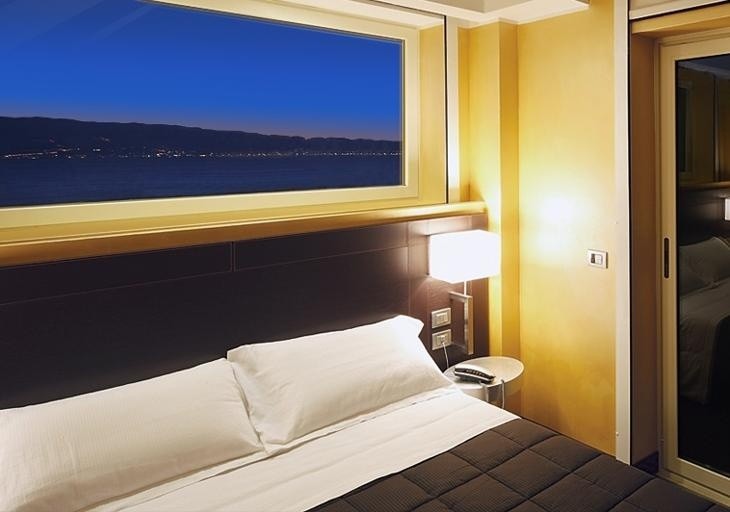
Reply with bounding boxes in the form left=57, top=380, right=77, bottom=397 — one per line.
left=0, top=314, right=730, bottom=511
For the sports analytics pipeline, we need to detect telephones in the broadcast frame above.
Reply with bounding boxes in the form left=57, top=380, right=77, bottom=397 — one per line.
left=454, top=364, right=496, bottom=383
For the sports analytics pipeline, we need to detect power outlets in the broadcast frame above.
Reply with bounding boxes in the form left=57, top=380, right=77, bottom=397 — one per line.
left=430, top=328, right=453, bottom=351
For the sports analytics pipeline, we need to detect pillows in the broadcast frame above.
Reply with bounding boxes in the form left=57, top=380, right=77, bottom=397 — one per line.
left=0, top=358, right=268, bottom=511
left=226, top=315, right=456, bottom=456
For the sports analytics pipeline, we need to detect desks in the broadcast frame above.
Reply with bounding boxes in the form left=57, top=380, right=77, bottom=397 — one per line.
left=443, top=354, right=525, bottom=410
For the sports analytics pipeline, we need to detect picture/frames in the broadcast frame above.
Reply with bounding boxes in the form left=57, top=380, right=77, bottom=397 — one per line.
left=0, top=0, right=423, bottom=234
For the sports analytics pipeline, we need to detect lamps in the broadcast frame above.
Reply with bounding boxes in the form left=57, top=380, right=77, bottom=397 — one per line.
left=427, top=229, right=502, bottom=355
